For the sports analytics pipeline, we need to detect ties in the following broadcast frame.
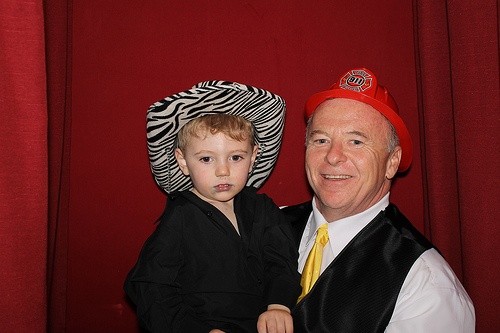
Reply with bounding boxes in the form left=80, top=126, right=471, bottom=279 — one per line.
left=293, top=222, right=330, bottom=305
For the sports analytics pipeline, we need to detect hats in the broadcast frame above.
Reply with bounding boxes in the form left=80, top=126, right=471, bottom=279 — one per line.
left=306, top=65, right=413, bottom=175
left=147, top=80, right=285, bottom=196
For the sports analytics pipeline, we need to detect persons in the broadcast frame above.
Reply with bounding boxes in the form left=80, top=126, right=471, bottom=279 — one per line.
left=122, top=80, right=302, bottom=333
left=279, top=67, right=477, bottom=333
